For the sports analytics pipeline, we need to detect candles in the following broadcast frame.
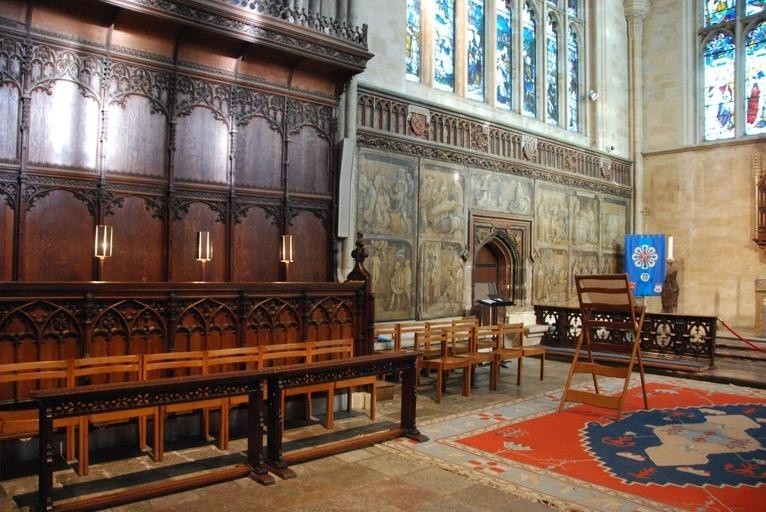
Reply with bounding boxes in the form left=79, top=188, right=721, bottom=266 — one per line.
left=667, top=235, right=674, bottom=260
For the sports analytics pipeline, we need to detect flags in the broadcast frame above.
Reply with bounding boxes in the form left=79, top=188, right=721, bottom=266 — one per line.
left=623, top=233, right=666, bottom=296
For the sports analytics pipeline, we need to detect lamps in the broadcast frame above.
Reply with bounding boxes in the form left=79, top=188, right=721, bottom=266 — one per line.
left=95, top=225, right=113, bottom=281
left=196, top=231, right=213, bottom=282
left=279, top=234, right=296, bottom=282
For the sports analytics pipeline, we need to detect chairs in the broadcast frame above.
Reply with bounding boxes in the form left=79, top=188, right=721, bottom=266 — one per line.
left=206, top=342, right=280, bottom=450
left=307, top=339, right=376, bottom=429
left=394, top=318, right=545, bottom=403
left=260, top=341, right=335, bottom=443
left=1, top=358, right=84, bottom=476
left=144, top=346, right=226, bottom=462
left=71, top=350, right=159, bottom=474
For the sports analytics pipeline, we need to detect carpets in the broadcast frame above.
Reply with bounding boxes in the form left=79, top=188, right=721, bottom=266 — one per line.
left=376, top=373, right=766, bottom=512
